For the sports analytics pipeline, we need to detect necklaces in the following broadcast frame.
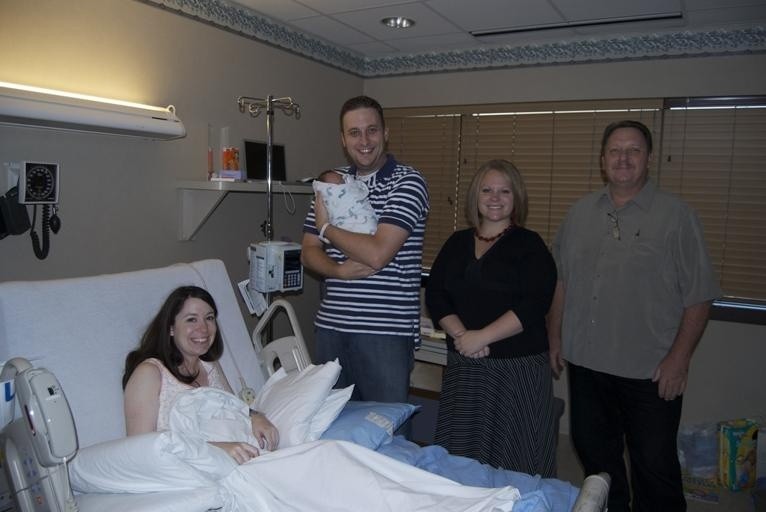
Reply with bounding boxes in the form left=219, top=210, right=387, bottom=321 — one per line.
left=476, top=226, right=512, bottom=243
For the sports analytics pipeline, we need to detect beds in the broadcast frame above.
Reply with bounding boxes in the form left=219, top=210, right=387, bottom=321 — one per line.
left=0, top=260, right=612, bottom=512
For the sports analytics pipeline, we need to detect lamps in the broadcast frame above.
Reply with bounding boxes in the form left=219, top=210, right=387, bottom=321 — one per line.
left=0, top=82, right=186, bottom=141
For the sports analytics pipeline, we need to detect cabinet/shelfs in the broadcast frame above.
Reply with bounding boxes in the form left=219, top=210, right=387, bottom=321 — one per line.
left=175, top=179, right=315, bottom=241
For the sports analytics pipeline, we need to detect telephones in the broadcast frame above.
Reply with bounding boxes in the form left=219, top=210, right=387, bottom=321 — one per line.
left=15, top=367, right=79, bottom=467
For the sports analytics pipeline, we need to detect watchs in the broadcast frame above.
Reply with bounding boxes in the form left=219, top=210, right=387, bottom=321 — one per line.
left=318, top=222, right=332, bottom=245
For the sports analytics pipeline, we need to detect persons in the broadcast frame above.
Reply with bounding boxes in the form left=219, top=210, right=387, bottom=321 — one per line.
left=317, top=169, right=345, bottom=184
left=424, top=159, right=558, bottom=480
left=299, top=94, right=430, bottom=441
left=121, top=286, right=280, bottom=466
left=548, top=120, right=724, bottom=512
